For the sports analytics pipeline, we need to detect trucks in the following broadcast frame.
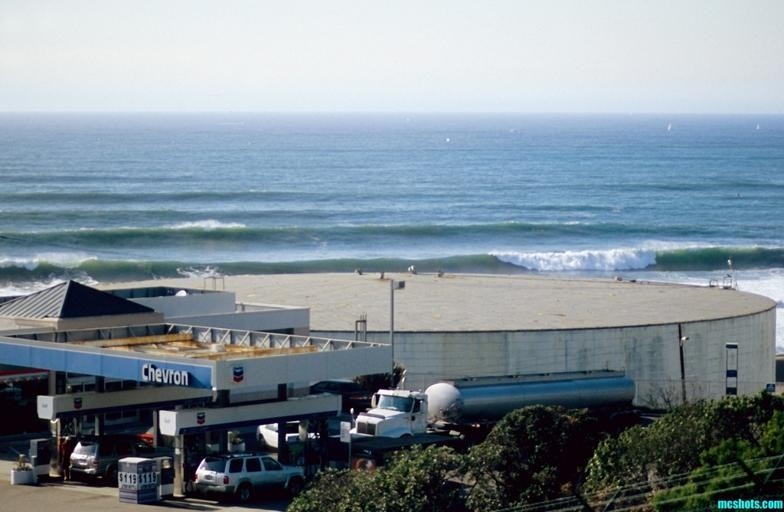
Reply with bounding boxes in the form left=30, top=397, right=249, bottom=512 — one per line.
left=387, top=278, right=406, bottom=391
left=349, top=368, right=637, bottom=466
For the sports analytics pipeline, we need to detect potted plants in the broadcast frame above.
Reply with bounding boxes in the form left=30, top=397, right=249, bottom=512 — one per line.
left=11, top=455, right=33, bottom=485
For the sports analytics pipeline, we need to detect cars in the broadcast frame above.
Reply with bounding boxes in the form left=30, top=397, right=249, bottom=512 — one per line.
left=194, top=453, right=306, bottom=501
left=68, top=433, right=170, bottom=486
